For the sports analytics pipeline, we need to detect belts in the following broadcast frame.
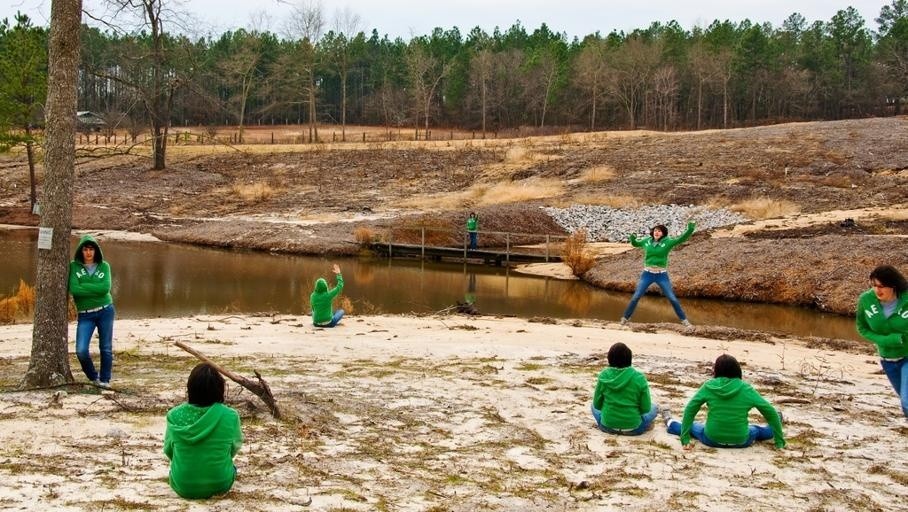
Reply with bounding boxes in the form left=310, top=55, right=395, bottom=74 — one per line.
left=78, top=304, right=108, bottom=314
left=878, top=356, right=904, bottom=362
left=644, top=270, right=666, bottom=274
left=612, top=429, right=633, bottom=432
left=314, top=320, right=332, bottom=326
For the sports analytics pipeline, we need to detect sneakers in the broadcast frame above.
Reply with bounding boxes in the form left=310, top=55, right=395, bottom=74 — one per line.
left=87, top=371, right=100, bottom=385
left=680, top=319, right=689, bottom=327
left=661, top=406, right=672, bottom=426
left=99, top=380, right=112, bottom=390
left=620, top=318, right=626, bottom=325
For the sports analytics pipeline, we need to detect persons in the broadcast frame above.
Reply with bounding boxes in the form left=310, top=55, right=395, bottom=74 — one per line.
left=464, top=272, right=479, bottom=307
left=467, top=210, right=481, bottom=249
left=620, top=216, right=696, bottom=326
left=854, top=264, right=908, bottom=421
left=66, top=234, right=118, bottom=396
left=660, top=353, right=789, bottom=450
left=589, top=340, right=658, bottom=437
left=309, top=263, right=347, bottom=328
left=164, top=362, right=242, bottom=501
